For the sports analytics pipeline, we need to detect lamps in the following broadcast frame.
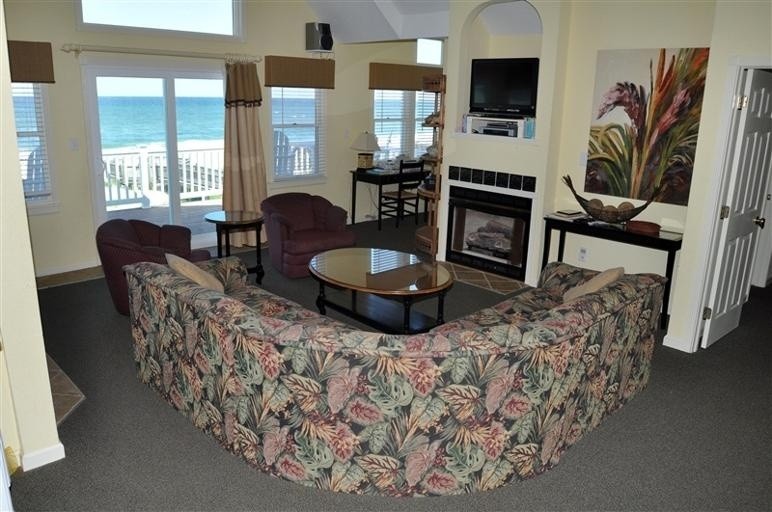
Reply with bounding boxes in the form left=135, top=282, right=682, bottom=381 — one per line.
left=349, top=130, right=380, bottom=169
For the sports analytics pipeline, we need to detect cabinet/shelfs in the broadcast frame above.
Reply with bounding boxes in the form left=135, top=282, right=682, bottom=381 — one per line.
left=415, top=74, right=446, bottom=256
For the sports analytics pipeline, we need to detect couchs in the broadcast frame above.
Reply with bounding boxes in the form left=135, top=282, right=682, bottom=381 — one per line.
left=122, top=254, right=670, bottom=497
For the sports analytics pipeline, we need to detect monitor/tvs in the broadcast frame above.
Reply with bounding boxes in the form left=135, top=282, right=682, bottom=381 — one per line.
left=470, top=57, right=539, bottom=119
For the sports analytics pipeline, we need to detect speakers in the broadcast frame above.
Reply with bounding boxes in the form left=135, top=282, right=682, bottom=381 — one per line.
left=305, top=22, right=333, bottom=53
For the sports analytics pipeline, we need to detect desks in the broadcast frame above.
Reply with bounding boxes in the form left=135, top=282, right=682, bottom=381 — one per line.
left=204, top=210, right=265, bottom=284
left=350, top=165, right=431, bottom=231
left=541, top=214, right=683, bottom=327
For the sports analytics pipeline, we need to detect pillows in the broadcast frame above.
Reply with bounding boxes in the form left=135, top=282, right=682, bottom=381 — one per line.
left=165, top=253, right=223, bottom=294
left=562, top=266, right=623, bottom=304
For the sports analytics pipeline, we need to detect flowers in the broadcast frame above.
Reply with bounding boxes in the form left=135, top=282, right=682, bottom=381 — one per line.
left=583, top=49, right=708, bottom=198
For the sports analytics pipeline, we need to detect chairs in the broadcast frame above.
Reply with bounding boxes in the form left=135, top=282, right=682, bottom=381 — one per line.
left=381, top=160, right=424, bottom=227
left=260, top=192, right=354, bottom=279
left=94, top=218, right=211, bottom=316
